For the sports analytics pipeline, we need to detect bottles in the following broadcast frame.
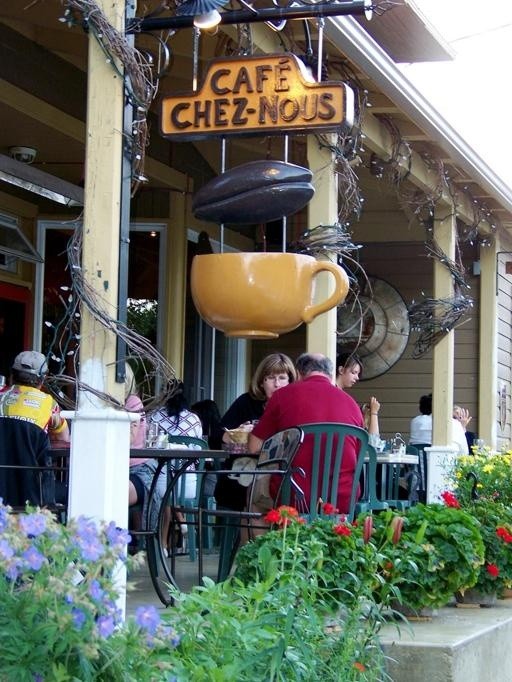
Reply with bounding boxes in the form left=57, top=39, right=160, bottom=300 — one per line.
left=148, top=430, right=167, bottom=449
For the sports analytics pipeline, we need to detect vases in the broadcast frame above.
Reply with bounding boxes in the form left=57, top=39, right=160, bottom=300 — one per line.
left=454, top=579, right=500, bottom=604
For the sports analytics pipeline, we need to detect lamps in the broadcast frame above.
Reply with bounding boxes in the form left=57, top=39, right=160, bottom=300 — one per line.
left=174, top=0, right=234, bottom=29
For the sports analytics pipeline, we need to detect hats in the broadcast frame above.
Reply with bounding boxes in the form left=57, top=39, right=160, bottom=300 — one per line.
left=13, top=350, right=50, bottom=378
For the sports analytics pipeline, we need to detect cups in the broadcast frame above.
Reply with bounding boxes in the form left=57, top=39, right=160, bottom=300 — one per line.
left=190, top=251, right=351, bottom=341
left=473, top=438, right=484, bottom=453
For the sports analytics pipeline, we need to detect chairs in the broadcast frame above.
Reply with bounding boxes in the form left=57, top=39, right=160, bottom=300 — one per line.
left=0, top=418, right=71, bottom=526
left=168, top=425, right=305, bottom=594
left=281, top=423, right=370, bottom=524
left=354, top=441, right=431, bottom=517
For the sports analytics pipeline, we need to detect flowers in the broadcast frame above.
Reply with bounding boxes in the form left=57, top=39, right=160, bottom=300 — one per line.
left=455, top=445, right=512, bottom=490
left=265, top=498, right=431, bottom=592
left=1, top=501, right=178, bottom=658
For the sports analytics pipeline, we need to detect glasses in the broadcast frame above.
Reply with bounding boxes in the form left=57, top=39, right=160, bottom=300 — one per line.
left=264, top=375, right=290, bottom=383
